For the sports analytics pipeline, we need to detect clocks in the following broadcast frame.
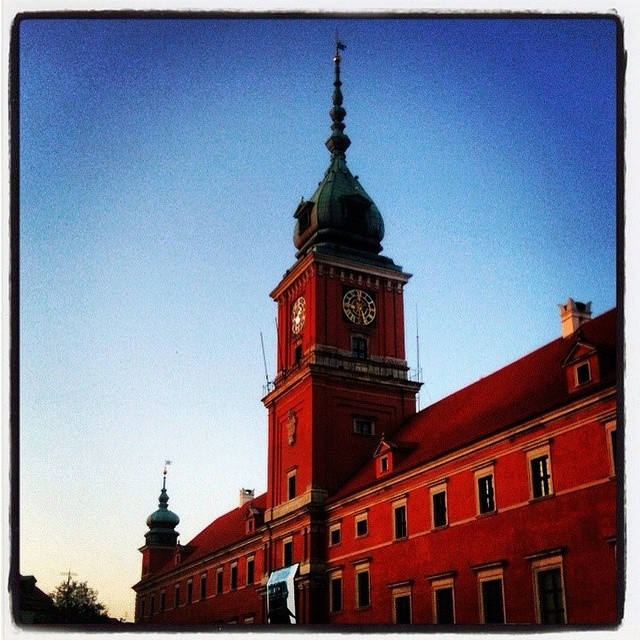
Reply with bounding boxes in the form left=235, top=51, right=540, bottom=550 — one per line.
left=342, top=289, right=377, bottom=326
left=290, top=296, right=306, bottom=335
